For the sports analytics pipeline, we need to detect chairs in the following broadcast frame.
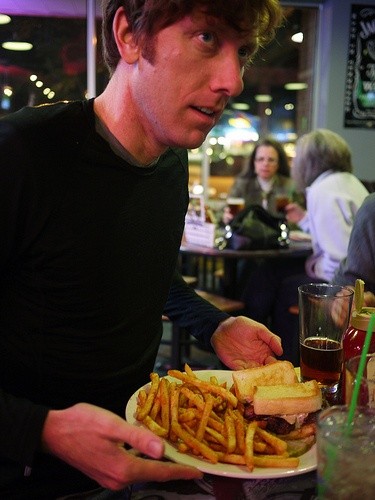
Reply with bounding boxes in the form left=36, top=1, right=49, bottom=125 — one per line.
left=157, top=290, right=244, bottom=371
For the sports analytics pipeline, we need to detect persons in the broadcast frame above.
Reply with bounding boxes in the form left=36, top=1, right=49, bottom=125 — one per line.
left=0, top=1, right=283, bottom=500
left=225, top=128, right=375, bottom=335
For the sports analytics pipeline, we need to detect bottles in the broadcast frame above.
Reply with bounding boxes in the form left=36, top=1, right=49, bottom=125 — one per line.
left=343, top=279, right=375, bottom=415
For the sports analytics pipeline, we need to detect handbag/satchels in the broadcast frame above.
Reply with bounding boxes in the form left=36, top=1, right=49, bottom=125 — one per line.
left=227, top=204, right=291, bottom=250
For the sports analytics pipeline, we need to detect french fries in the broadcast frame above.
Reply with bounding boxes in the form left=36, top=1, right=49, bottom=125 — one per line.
left=134, top=364, right=299, bottom=472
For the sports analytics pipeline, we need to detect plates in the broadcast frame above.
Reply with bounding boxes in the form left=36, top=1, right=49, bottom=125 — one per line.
left=124, top=369, right=345, bottom=478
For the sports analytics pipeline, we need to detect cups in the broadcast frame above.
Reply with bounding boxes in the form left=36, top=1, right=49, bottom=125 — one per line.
left=226, top=196, right=245, bottom=216
left=345, top=353, right=374, bottom=413
left=296, top=282, right=354, bottom=394
left=275, top=190, right=291, bottom=214
left=315, top=402, right=375, bottom=500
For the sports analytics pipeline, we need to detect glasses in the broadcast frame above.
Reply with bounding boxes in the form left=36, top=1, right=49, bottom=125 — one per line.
left=253, top=156, right=280, bottom=167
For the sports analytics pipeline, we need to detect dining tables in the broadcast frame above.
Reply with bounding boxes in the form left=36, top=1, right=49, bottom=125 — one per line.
left=179, top=224, right=312, bottom=299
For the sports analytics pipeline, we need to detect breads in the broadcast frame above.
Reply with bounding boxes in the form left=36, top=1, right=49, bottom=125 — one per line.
left=233, top=360, right=322, bottom=414
